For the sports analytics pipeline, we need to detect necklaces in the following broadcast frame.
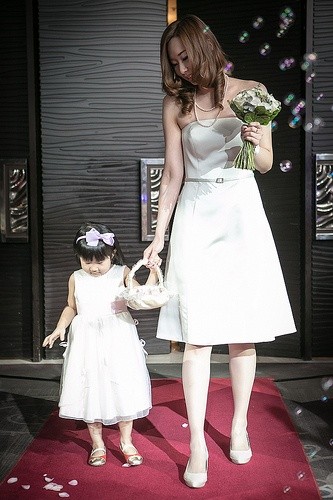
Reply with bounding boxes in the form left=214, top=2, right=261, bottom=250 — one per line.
left=194, top=74, right=229, bottom=127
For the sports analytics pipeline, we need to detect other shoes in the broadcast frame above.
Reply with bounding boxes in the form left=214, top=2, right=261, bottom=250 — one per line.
left=120, top=446, right=143, bottom=465
left=88, top=446, right=106, bottom=466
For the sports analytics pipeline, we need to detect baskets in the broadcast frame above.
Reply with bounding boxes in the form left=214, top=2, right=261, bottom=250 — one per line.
left=121, top=261, right=169, bottom=310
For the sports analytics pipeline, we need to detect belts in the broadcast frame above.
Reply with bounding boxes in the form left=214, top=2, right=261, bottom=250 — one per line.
left=185, top=174, right=255, bottom=183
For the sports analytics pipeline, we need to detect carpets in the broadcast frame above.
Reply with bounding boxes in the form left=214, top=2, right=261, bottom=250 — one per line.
left=0, top=378, right=321, bottom=500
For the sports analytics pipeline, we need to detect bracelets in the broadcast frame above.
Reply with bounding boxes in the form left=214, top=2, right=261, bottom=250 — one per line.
left=253, top=145, right=259, bottom=154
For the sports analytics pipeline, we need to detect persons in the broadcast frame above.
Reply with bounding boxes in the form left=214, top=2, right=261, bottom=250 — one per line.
left=142, top=14, right=297, bottom=487
left=43, top=223, right=164, bottom=467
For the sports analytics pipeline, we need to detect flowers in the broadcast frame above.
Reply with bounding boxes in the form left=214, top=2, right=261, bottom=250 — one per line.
left=225, top=85, right=282, bottom=122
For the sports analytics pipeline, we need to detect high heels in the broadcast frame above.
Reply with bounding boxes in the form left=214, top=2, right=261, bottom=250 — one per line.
left=182, top=447, right=209, bottom=487
left=229, top=430, right=252, bottom=465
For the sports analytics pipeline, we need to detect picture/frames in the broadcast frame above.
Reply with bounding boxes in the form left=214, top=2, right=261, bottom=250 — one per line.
left=315, top=153, right=333, bottom=240
left=0, top=159, right=29, bottom=242
left=140, top=158, right=171, bottom=242
